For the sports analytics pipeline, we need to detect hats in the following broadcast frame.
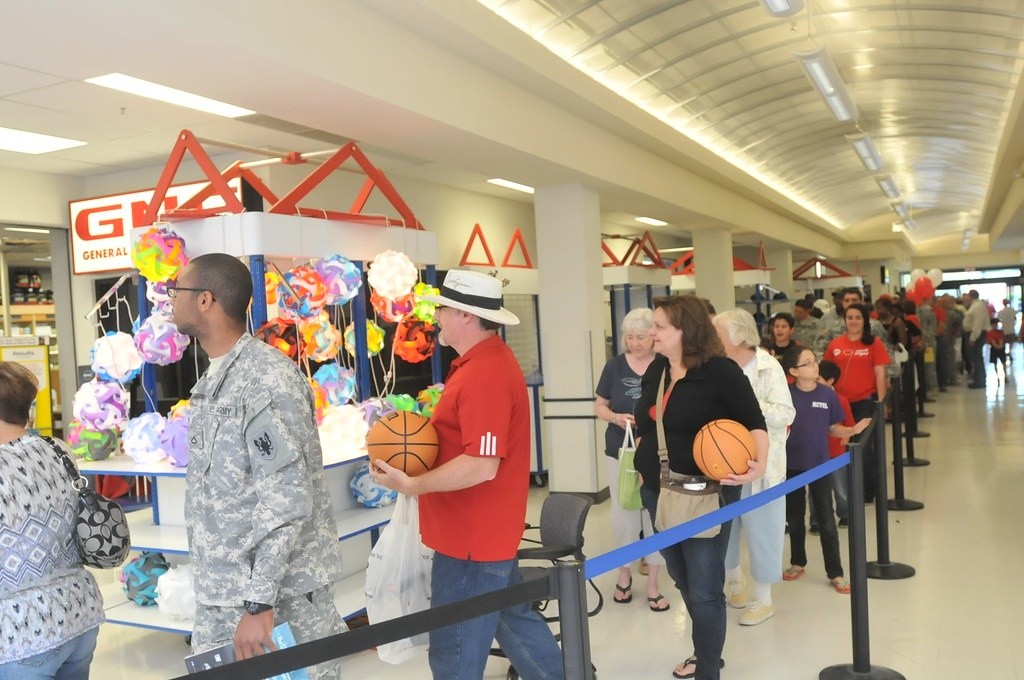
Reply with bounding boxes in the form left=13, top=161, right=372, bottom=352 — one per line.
left=832, top=289, right=844, bottom=298
left=813, top=298, right=830, bottom=314
left=423, top=269, right=520, bottom=325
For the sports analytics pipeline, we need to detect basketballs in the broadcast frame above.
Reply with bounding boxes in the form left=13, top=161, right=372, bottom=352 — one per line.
left=691, top=418, right=757, bottom=481
left=367, top=410, right=439, bottom=477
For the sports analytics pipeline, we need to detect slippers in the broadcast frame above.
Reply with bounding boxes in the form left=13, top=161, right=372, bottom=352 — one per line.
left=831, top=578, right=851, bottom=594
left=614, top=574, right=633, bottom=604
left=783, top=568, right=807, bottom=580
left=647, top=594, right=671, bottom=612
left=672, top=657, right=725, bottom=678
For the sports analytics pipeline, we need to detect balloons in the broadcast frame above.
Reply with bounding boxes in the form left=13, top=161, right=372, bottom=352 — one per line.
left=903, top=267, right=943, bottom=304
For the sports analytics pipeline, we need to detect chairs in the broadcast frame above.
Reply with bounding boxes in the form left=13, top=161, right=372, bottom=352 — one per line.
left=489, top=493, right=603, bottom=680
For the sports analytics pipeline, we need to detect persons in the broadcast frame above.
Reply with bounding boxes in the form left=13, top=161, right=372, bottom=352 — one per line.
left=998, top=298, right=1016, bottom=361
left=700, top=299, right=796, bottom=627
left=783, top=347, right=871, bottom=595
left=823, top=303, right=890, bottom=505
left=633, top=297, right=769, bottom=680
left=786, top=285, right=991, bottom=420
left=367, top=269, right=565, bottom=680
left=0, top=360, right=134, bottom=679
left=770, top=310, right=798, bottom=536
left=170, top=253, right=350, bottom=680
left=594, top=307, right=671, bottom=612
left=986, top=317, right=1009, bottom=384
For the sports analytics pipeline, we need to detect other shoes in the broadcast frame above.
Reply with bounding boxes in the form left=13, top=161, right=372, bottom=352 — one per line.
left=864, top=496, right=873, bottom=503
left=968, top=383, right=987, bottom=388
left=809, top=525, right=820, bottom=536
left=638, top=557, right=650, bottom=575
left=839, top=517, right=850, bottom=528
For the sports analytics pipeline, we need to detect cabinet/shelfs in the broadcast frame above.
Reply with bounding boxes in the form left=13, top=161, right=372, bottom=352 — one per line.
left=0, top=316, right=60, bottom=407
left=74, top=442, right=395, bottom=645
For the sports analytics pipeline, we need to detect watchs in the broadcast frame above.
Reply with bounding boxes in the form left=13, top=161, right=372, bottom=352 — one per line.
left=612, top=413, right=617, bottom=425
left=243, top=600, right=273, bottom=615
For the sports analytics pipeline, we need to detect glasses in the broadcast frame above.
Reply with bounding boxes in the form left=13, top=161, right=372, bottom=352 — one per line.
left=795, top=356, right=820, bottom=369
left=434, top=304, right=449, bottom=313
left=167, top=286, right=216, bottom=302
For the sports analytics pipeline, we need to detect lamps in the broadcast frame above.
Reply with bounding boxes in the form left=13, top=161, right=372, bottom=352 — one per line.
left=961, top=227, right=972, bottom=251
left=891, top=201, right=916, bottom=230
left=844, top=131, right=883, bottom=172
left=760, top=0, right=804, bottom=18
left=791, top=44, right=858, bottom=123
left=875, top=175, right=900, bottom=199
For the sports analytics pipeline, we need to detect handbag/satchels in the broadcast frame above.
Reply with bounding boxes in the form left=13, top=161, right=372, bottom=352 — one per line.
left=617, top=419, right=645, bottom=510
left=37, top=435, right=131, bottom=569
left=654, top=467, right=724, bottom=538
left=364, top=491, right=435, bottom=665
left=894, top=342, right=908, bottom=363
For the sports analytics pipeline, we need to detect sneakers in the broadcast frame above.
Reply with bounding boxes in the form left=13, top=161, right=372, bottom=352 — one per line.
left=740, top=601, right=775, bottom=626
left=726, top=574, right=749, bottom=608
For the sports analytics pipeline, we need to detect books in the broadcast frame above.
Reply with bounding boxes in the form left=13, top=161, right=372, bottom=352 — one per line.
left=184, top=622, right=310, bottom=680
